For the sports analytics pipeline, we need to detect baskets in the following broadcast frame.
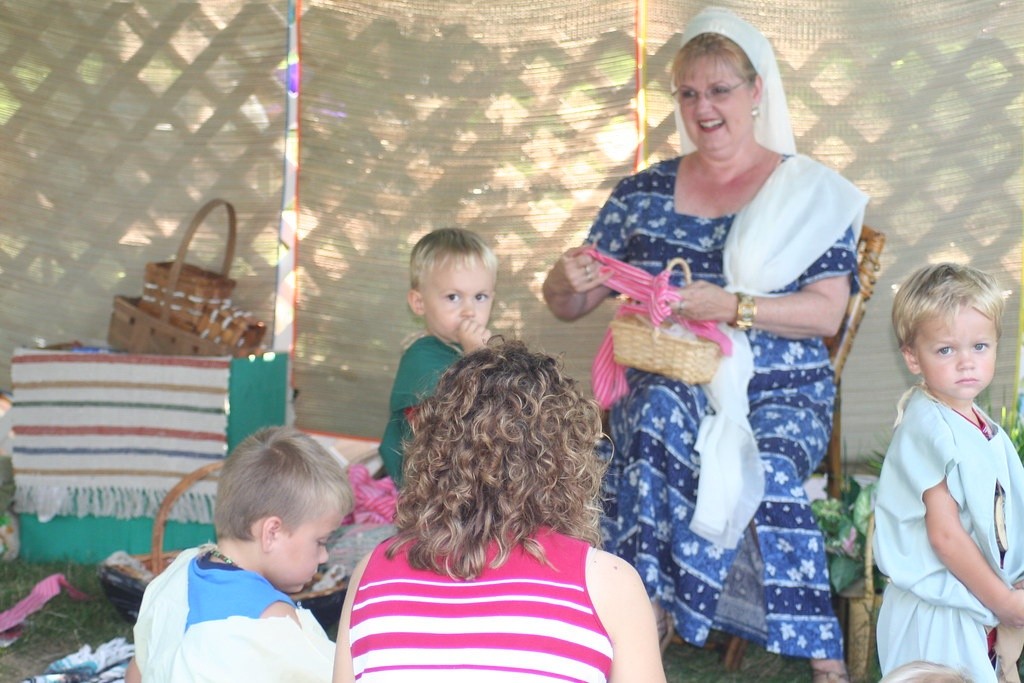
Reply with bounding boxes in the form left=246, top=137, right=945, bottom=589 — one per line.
left=97, top=461, right=350, bottom=636
left=109, top=197, right=270, bottom=360
left=611, top=255, right=719, bottom=383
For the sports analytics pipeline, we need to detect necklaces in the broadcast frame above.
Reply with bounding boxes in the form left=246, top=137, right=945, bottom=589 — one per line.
left=209, top=548, right=240, bottom=570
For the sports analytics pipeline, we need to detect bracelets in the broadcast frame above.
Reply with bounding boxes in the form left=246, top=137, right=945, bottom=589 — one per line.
left=727, top=291, right=757, bottom=335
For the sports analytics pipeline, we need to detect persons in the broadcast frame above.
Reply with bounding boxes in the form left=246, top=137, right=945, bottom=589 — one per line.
left=328, top=333, right=673, bottom=683
left=533, top=1, right=871, bottom=683
left=866, top=258, right=1024, bottom=683
left=378, top=228, right=511, bottom=495
left=121, top=422, right=345, bottom=683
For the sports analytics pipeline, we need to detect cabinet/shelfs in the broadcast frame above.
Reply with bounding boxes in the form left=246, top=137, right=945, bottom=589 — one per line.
left=12, top=343, right=286, bottom=561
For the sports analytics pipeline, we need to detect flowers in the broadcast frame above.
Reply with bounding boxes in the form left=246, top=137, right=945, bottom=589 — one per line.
left=805, top=479, right=875, bottom=596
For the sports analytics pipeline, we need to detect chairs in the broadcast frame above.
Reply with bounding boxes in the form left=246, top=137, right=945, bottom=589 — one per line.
left=669, top=222, right=881, bottom=672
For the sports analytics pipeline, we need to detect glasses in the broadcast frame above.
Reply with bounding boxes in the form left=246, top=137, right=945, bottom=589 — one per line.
left=673, top=78, right=750, bottom=106
